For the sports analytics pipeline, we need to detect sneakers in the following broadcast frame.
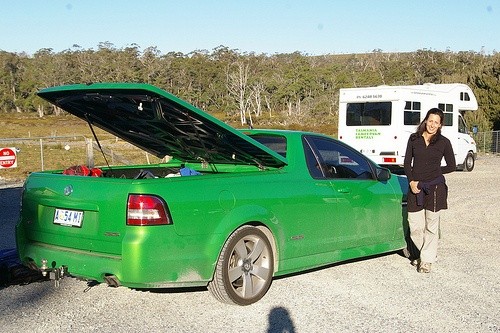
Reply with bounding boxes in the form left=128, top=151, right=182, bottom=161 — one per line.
left=417, top=260, right=432, bottom=273
left=413, top=256, right=438, bottom=265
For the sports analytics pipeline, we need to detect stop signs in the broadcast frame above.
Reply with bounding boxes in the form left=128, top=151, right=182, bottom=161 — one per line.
left=0, top=146, right=17, bottom=169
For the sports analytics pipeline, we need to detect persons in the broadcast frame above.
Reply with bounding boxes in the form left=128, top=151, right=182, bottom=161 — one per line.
left=404, top=108, right=456, bottom=274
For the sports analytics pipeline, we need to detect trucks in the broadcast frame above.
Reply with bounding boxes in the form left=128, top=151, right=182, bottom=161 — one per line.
left=337, top=82, right=480, bottom=173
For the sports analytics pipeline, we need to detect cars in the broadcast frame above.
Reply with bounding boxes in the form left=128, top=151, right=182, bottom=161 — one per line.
left=15, top=84, right=440, bottom=306
